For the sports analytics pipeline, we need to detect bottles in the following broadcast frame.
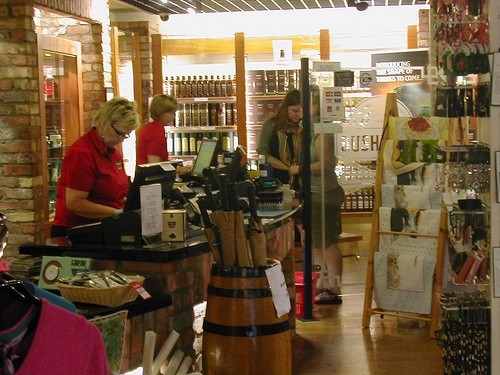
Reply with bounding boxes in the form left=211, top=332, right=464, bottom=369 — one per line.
left=192, top=76, right=198, bottom=98
left=202, top=133, right=209, bottom=140
left=175, top=76, right=180, bottom=98
left=340, top=193, right=374, bottom=212
left=196, top=133, right=202, bottom=155
left=189, top=133, right=196, bottom=155
left=175, top=102, right=238, bottom=127
left=182, top=133, right=189, bottom=156
left=49, top=126, right=61, bottom=148
left=218, top=153, right=224, bottom=164
left=45, top=73, right=54, bottom=100
left=185, top=76, right=191, bottom=98
left=224, top=153, right=235, bottom=165
left=222, top=132, right=230, bottom=152
left=180, top=76, right=186, bottom=98
left=204, top=75, right=234, bottom=97
left=163, top=76, right=168, bottom=95
left=212, top=133, right=218, bottom=140
left=173, top=133, right=181, bottom=156
left=198, top=75, right=204, bottom=97
left=50, top=159, right=58, bottom=186
left=167, top=133, right=173, bottom=156
left=169, top=76, right=175, bottom=98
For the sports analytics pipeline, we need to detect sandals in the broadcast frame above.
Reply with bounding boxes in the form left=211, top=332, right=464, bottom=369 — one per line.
left=315, top=287, right=342, bottom=303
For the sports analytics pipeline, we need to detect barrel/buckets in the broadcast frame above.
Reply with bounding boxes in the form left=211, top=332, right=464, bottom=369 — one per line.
left=202, top=258, right=292, bottom=375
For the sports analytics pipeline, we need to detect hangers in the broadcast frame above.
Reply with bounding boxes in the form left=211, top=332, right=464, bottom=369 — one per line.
left=0, top=269, right=41, bottom=305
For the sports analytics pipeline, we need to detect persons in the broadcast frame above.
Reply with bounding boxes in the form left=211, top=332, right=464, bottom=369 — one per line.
left=288, top=83, right=345, bottom=304
left=136, top=94, right=178, bottom=169
left=50, top=97, right=141, bottom=247
left=256, top=88, right=304, bottom=239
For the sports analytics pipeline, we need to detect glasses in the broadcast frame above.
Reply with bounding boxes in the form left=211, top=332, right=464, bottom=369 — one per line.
left=111, top=124, right=131, bottom=139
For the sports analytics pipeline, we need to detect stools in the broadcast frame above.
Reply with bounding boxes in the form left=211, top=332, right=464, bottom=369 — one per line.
left=337, top=233, right=363, bottom=256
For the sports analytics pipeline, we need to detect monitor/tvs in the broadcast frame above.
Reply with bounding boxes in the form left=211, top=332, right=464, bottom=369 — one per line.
left=123, top=161, right=178, bottom=212
left=191, top=139, right=220, bottom=177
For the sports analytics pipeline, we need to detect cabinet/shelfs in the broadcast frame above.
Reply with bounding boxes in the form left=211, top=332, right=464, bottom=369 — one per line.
left=245, top=95, right=285, bottom=159
left=169, top=97, right=238, bottom=161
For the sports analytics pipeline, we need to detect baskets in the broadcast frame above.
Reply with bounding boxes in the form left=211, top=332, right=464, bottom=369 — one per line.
left=56, top=273, right=145, bottom=307
